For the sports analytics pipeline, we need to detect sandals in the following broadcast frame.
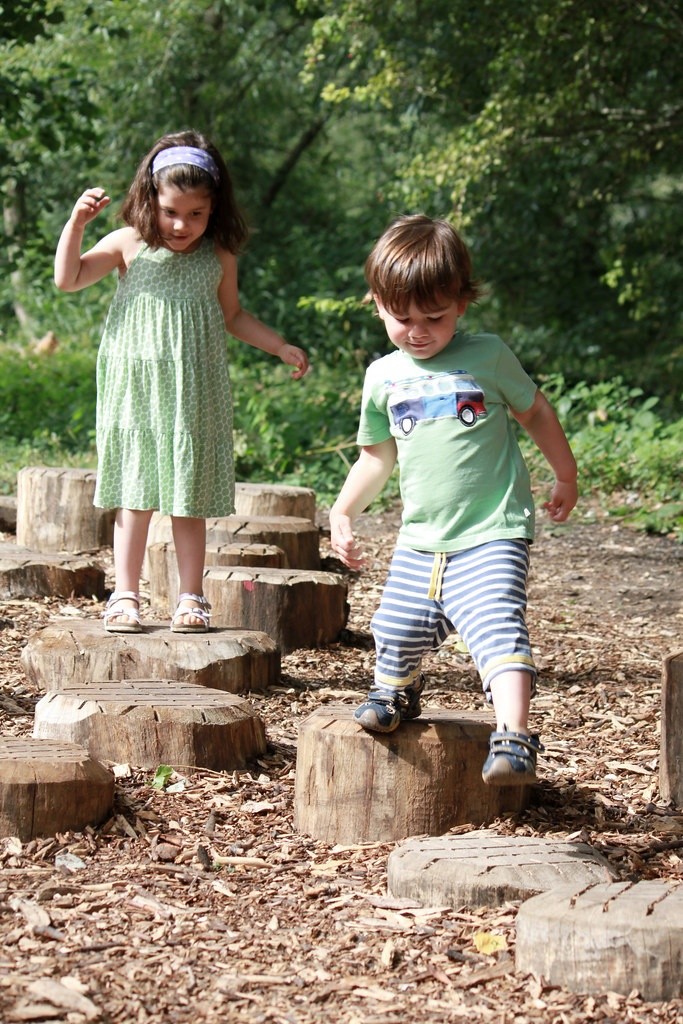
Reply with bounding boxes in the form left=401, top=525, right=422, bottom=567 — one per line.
left=103, top=591, right=143, bottom=633
left=170, top=593, right=212, bottom=632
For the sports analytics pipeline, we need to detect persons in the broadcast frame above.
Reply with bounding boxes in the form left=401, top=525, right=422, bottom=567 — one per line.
left=330, top=215, right=579, bottom=783
left=55, top=130, right=309, bottom=633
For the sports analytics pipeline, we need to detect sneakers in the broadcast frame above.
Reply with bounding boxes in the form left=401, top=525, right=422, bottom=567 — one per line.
left=482, top=731, right=545, bottom=786
left=354, top=671, right=426, bottom=733
